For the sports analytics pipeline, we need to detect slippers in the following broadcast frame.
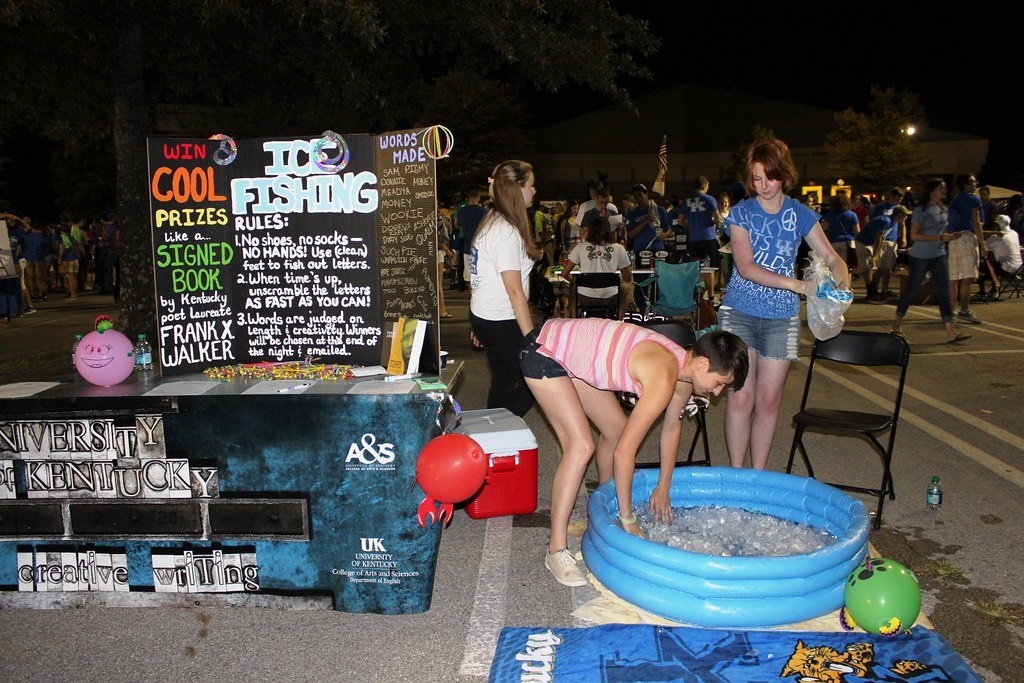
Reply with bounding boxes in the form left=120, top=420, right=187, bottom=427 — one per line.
left=947, top=331, right=971, bottom=344
left=890, top=327, right=909, bottom=344
left=440, top=312, right=453, bottom=319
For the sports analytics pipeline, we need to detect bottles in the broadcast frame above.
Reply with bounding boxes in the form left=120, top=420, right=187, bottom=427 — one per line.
left=704, top=255, right=712, bottom=268
left=135, top=333, right=154, bottom=371
left=926, top=475, right=943, bottom=513
left=71, top=334, right=83, bottom=370
left=629, top=250, right=636, bottom=263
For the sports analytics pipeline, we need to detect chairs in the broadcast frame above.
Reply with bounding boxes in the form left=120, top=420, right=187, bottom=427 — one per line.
left=992, top=260, right=1024, bottom=301
left=575, top=272, right=621, bottom=321
left=783, top=329, right=910, bottom=530
left=615, top=320, right=711, bottom=468
left=631, top=259, right=702, bottom=332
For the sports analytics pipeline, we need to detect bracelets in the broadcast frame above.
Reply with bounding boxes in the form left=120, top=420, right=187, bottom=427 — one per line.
left=616, top=514, right=637, bottom=524
left=940, top=235, right=944, bottom=242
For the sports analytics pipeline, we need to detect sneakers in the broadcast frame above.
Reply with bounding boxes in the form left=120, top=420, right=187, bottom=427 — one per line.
left=544, top=546, right=586, bottom=586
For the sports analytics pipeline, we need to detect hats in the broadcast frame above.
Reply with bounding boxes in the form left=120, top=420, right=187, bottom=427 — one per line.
left=630, top=184, right=647, bottom=194
left=892, top=205, right=913, bottom=215
left=997, top=215, right=1011, bottom=232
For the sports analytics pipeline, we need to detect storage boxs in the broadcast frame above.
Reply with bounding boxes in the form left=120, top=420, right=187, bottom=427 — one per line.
left=449, top=408, right=539, bottom=520
left=894, top=270, right=960, bottom=305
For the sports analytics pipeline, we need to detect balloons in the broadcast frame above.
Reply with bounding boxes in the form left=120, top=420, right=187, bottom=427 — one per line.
left=75, top=328, right=136, bottom=386
left=844, top=558, right=921, bottom=638
left=416, top=433, right=487, bottom=504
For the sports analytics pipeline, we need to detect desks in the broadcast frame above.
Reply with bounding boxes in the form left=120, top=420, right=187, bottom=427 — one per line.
left=554, top=267, right=718, bottom=298
left=0, top=359, right=465, bottom=616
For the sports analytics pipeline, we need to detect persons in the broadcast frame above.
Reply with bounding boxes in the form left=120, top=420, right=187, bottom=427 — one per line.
left=665, top=177, right=730, bottom=307
left=796, top=186, right=913, bottom=304
left=436, top=190, right=490, bottom=319
left=0, top=213, right=120, bottom=314
left=889, top=172, right=1024, bottom=343
left=532, top=183, right=671, bottom=320
left=517, top=318, right=749, bottom=586
left=716, top=134, right=849, bottom=469
left=471, top=159, right=543, bottom=419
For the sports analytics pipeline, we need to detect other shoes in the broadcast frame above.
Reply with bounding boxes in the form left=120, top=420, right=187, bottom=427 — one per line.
left=23, top=306, right=36, bottom=314
left=989, top=288, right=1001, bottom=299
left=65, top=295, right=77, bottom=301
left=866, top=291, right=882, bottom=301
left=881, top=289, right=897, bottom=297
left=84, top=285, right=93, bottom=290
left=957, top=310, right=982, bottom=324
left=31, top=296, right=43, bottom=302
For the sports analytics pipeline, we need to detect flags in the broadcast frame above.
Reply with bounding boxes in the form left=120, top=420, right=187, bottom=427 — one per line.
left=651, top=134, right=667, bottom=197
